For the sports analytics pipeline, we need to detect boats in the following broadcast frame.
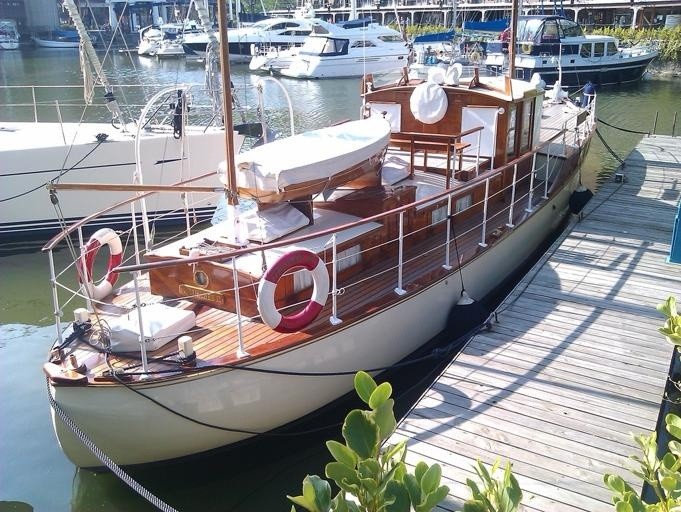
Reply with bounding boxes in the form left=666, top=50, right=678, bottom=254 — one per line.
left=0, top=83, right=278, bottom=252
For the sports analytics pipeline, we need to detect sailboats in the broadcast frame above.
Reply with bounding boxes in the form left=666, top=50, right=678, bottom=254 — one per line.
left=38, top=0, right=600, bottom=471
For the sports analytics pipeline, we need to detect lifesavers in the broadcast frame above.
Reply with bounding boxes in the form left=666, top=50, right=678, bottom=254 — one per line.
left=257, top=248, right=330, bottom=333
left=78, top=228, right=123, bottom=299
